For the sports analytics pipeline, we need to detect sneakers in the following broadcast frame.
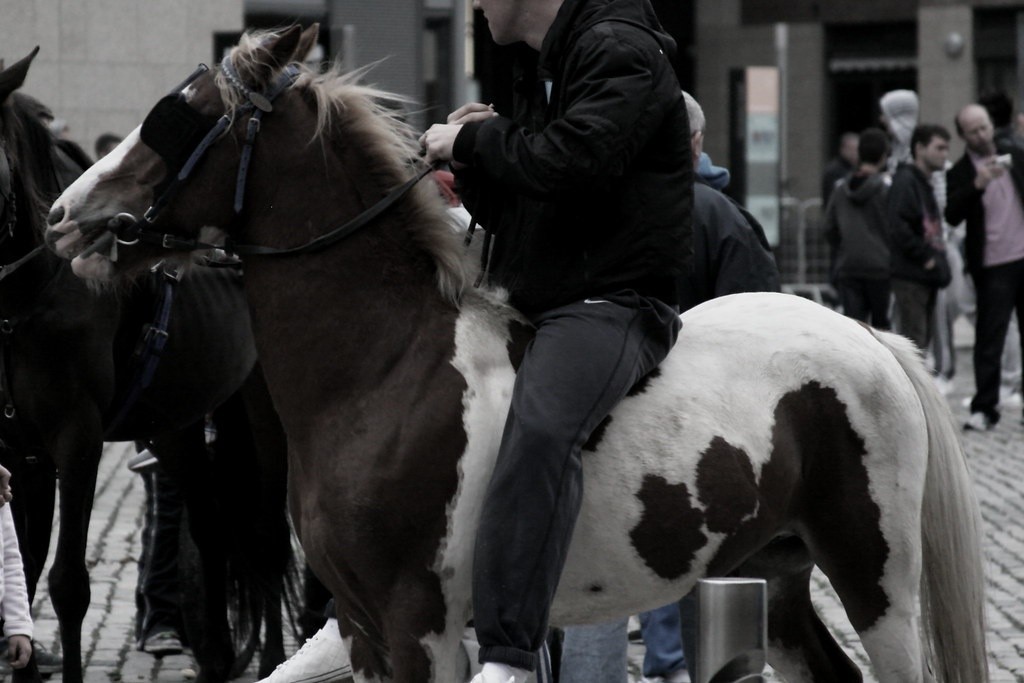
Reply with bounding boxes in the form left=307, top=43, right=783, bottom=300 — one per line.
left=256, top=628, right=352, bottom=683
left=465, top=663, right=537, bottom=683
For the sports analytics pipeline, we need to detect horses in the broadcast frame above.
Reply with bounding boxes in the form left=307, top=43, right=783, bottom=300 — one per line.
left=42, top=23, right=993, bottom=683
left=0, top=46, right=328, bottom=682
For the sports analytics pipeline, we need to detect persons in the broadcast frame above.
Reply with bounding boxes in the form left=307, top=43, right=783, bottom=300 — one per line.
left=128, top=440, right=187, bottom=654
left=640, top=90, right=782, bottom=683
left=94, top=133, right=122, bottom=160
left=0, top=465, right=34, bottom=682
left=418, top=0, right=698, bottom=683
left=814, top=85, right=1024, bottom=436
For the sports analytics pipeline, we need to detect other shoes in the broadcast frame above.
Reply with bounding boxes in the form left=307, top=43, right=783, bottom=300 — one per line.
left=628, top=630, right=644, bottom=643
left=935, top=377, right=952, bottom=395
left=136, top=631, right=184, bottom=653
left=963, top=412, right=999, bottom=430
left=0, top=638, right=63, bottom=673
